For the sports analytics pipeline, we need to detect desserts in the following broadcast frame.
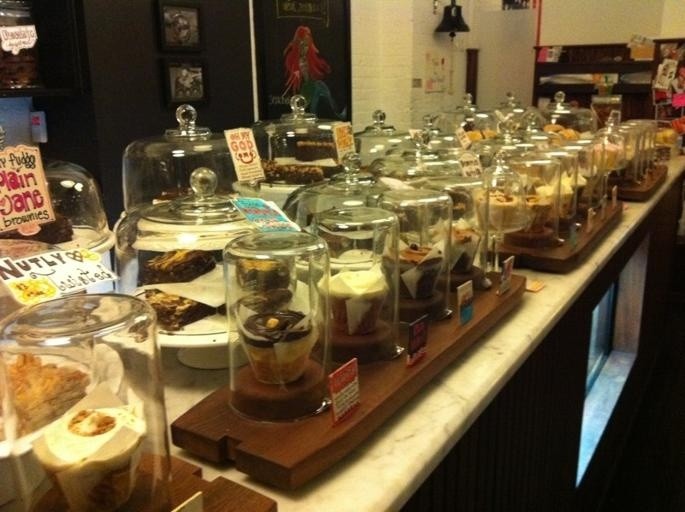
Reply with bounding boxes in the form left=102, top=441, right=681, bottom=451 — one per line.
left=1, top=124, right=675, bottom=512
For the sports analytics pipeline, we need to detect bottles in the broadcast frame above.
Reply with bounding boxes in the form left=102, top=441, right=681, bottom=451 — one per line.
left=0, top=90, right=661, bottom=510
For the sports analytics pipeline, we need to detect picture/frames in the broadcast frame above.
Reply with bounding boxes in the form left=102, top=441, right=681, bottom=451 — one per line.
left=155, top=1, right=211, bottom=108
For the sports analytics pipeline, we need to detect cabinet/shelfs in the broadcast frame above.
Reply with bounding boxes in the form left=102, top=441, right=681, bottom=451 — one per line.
left=534, top=39, right=684, bottom=120
